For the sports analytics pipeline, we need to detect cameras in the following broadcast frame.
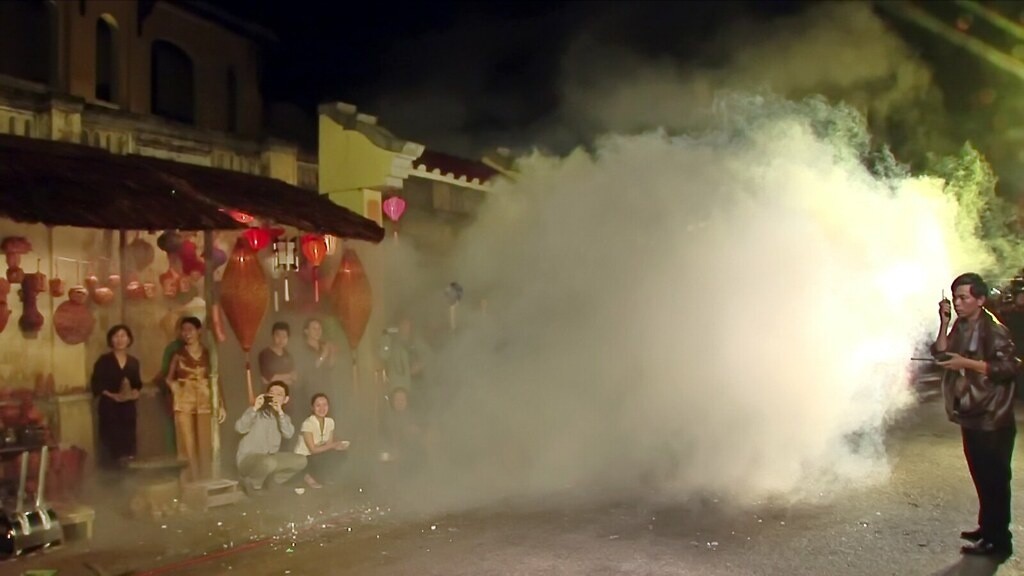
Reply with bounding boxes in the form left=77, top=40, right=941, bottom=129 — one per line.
left=264, top=396, right=273, bottom=402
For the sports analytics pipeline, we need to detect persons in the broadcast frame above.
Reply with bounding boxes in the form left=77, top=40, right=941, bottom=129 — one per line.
left=301, top=319, right=336, bottom=402
left=162, top=314, right=187, bottom=452
left=258, top=321, right=300, bottom=417
left=931, top=272, right=1023, bottom=553
left=91, top=325, right=144, bottom=471
left=380, top=387, right=425, bottom=464
left=235, top=381, right=308, bottom=500
left=294, top=393, right=352, bottom=488
left=166, top=317, right=228, bottom=483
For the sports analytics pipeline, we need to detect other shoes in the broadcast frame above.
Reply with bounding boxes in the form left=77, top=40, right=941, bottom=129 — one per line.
left=245, top=476, right=264, bottom=496
left=304, top=473, right=323, bottom=489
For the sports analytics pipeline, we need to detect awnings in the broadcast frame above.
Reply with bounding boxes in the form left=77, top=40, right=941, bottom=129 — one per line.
left=1, top=128, right=388, bottom=245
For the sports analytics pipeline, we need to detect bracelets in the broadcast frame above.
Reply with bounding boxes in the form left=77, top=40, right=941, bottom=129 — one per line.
left=978, top=359, right=984, bottom=373
left=320, top=356, right=326, bottom=361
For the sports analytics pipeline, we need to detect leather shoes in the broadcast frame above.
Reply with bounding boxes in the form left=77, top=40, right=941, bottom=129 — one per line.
left=962, top=538, right=1012, bottom=555
left=960, top=528, right=982, bottom=540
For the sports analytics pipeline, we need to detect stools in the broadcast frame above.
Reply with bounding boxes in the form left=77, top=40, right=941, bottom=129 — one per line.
left=188, top=477, right=239, bottom=513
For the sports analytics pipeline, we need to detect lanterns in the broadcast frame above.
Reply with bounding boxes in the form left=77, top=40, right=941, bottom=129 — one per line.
left=383, top=196, right=407, bottom=231
left=0, top=224, right=374, bottom=405
